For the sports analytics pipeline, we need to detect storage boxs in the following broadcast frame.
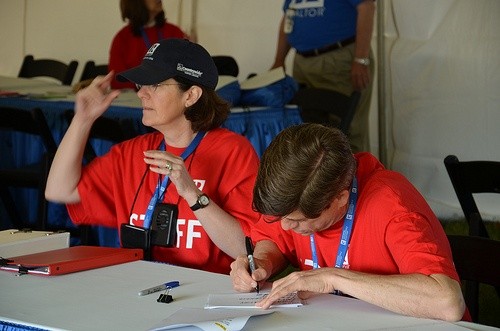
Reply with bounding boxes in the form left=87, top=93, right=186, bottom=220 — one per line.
left=0, top=229, right=71, bottom=259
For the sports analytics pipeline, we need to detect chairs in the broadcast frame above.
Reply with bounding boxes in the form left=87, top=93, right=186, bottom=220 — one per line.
left=444, top=154, right=500, bottom=239
left=80, top=61, right=110, bottom=82
left=210, top=54, right=239, bottom=77
left=17, top=55, right=79, bottom=87
left=445, top=235, right=500, bottom=324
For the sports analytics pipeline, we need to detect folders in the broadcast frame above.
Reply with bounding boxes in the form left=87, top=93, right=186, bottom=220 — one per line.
left=0, top=246, right=144, bottom=275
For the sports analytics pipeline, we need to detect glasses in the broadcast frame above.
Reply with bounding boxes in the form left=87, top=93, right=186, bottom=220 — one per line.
left=136, top=83, right=184, bottom=93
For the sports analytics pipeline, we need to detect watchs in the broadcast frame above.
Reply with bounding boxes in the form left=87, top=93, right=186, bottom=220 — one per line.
left=354, top=57, right=371, bottom=66
left=190, top=194, right=209, bottom=211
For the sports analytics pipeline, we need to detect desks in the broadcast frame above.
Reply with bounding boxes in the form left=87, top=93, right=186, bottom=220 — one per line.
left=0, top=259, right=500, bottom=331
left=0, top=74, right=305, bottom=248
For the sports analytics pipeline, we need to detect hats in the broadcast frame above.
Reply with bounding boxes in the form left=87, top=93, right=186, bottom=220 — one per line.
left=116, top=38, right=218, bottom=89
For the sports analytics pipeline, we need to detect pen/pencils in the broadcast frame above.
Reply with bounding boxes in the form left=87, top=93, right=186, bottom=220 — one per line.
left=245, top=236, right=259, bottom=294
left=137, top=280, right=180, bottom=296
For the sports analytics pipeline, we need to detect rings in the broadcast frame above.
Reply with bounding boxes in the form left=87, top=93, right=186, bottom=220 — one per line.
left=167, top=161, right=173, bottom=169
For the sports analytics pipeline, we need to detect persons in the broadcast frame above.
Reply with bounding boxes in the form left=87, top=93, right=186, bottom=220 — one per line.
left=228, top=125, right=473, bottom=322
left=269, top=0, right=378, bottom=156
left=45, top=38, right=261, bottom=276
left=109, top=1, right=189, bottom=90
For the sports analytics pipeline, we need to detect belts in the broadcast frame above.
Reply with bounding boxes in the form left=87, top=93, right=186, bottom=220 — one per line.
left=297, top=37, right=356, bottom=58
